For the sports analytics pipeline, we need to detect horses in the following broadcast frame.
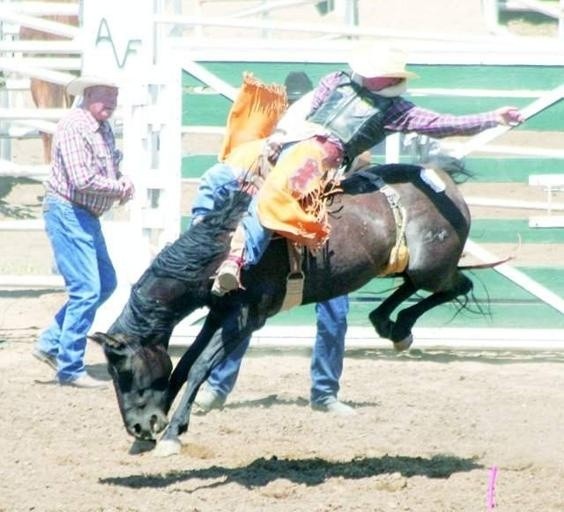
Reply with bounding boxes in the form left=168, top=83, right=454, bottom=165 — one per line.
left=86, top=136, right=491, bottom=459
left=15, top=9, right=82, bottom=168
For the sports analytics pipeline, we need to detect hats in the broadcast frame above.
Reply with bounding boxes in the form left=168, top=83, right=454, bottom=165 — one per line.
left=63, top=53, right=127, bottom=98
left=344, top=41, right=421, bottom=82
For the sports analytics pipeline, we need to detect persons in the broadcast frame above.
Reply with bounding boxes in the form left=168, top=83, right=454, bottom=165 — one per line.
left=194, top=135, right=364, bottom=415
left=28, top=62, right=132, bottom=387
left=187, top=60, right=529, bottom=300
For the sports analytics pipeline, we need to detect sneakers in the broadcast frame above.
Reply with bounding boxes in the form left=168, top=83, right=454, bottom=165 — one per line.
left=71, top=372, right=109, bottom=388
left=310, top=399, right=355, bottom=414
left=191, top=388, right=226, bottom=413
left=208, top=263, right=239, bottom=299
left=29, top=348, right=60, bottom=372
